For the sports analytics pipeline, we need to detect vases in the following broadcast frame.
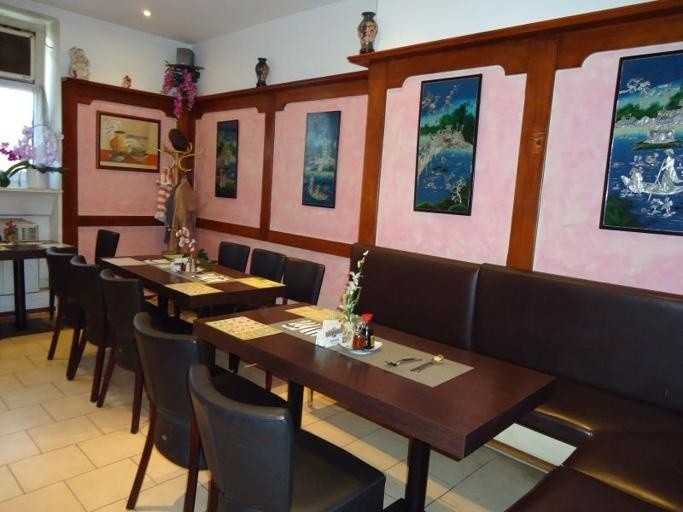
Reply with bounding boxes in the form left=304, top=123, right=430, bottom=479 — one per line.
left=255, top=58, right=269, bottom=88
left=110, top=131, right=129, bottom=153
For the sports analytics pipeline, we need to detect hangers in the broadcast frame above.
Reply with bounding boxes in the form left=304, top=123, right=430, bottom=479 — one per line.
left=156, top=142, right=204, bottom=173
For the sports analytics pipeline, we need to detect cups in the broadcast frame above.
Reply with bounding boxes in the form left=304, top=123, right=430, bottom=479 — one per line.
left=132, top=147, right=145, bottom=157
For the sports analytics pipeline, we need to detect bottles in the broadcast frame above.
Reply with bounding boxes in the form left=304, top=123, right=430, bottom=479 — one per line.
left=176, top=259, right=192, bottom=272
left=350, top=320, right=374, bottom=348
left=254, top=56, right=269, bottom=86
left=109, top=130, right=127, bottom=154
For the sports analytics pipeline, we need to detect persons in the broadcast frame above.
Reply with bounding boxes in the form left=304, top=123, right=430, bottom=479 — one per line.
left=662, top=149, right=683, bottom=191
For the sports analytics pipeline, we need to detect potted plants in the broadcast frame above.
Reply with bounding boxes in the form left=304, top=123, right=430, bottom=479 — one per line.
left=125, top=136, right=149, bottom=161
left=108, top=151, right=127, bottom=162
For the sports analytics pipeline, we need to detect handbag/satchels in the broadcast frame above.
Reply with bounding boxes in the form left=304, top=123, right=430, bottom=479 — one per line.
left=153, top=181, right=173, bottom=222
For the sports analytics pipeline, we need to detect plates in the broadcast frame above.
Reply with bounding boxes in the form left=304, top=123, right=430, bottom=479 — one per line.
left=129, top=154, right=148, bottom=162
left=171, top=266, right=206, bottom=277
left=338, top=338, right=384, bottom=355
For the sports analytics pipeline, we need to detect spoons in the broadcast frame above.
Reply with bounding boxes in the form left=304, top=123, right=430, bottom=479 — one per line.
left=411, top=351, right=446, bottom=373
left=385, top=357, right=419, bottom=367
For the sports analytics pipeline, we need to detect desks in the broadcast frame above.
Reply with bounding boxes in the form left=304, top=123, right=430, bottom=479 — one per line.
left=0, top=239, right=74, bottom=340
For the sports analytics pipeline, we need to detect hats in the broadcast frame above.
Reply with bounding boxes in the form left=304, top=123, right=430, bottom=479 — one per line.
left=169, top=129, right=188, bottom=151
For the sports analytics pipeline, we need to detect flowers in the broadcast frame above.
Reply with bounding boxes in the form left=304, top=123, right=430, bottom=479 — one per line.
left=0, top=124, right=71, bottom=188
left=163, top=65, right=200, bottom=118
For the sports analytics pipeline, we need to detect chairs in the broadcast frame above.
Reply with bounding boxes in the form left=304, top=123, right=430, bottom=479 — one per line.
left=217, top=241, right=325, bottom=303
left=126, top=313, right=286, bottom=512
left=188, top=365, right=386, bottom=511
left=45, top=230, right=148, bottom=434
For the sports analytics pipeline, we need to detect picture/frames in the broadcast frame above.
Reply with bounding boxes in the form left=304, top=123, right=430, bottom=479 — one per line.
left=302, top=111, right=341, bottom=208
left=599, top=49, right=683, bottom=236
left=215, top=120, right=238, bottom=199
left=96, top=110, right=161, bottom=173
left=414, top=74, right=482, bottom=216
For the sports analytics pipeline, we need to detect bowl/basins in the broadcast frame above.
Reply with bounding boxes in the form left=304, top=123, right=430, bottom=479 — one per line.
left=108, top=153, right=126, bottom=161
